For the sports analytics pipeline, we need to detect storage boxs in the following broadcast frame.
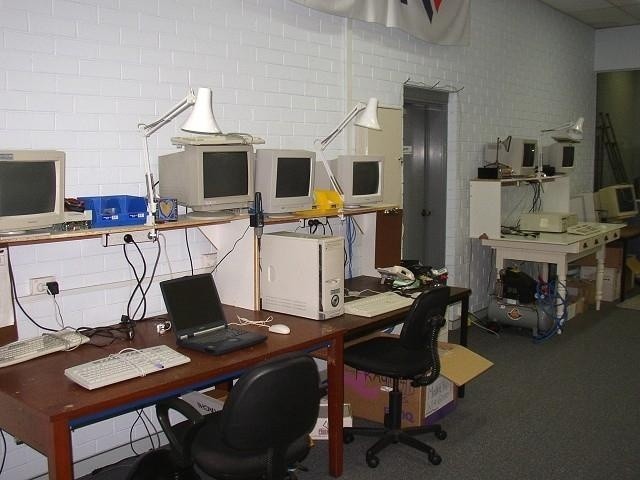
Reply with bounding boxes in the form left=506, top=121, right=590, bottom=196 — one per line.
left=580, top=242, right=625, bottom=267
left=343, top=330, right=494, bottom=430
left=580, top=266, right=623, bottom=303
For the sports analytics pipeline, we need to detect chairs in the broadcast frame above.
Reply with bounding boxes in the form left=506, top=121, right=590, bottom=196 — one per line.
left=340, top=285, right=448, bottom=466
left=155, top=356, right=322, bottom=479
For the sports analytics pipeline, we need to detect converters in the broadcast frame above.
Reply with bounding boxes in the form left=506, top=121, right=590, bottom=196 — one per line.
left=349, top=291, right=362, bottom=297
left=47, top=282, right=59, bottom=295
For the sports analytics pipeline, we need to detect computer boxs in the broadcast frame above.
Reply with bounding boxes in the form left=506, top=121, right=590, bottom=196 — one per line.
left=261, top=231, right=346, bottom=322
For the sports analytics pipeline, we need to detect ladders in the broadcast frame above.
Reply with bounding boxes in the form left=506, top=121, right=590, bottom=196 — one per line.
left=594, top=111, right=628, bottom=189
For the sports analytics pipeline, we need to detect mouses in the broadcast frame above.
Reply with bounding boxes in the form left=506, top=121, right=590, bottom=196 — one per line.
left=411, top=292, right=421, bottom=298
left=269, top=324, right=291, bottom=335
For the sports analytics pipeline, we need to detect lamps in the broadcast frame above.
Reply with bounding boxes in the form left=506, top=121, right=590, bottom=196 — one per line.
left=538, top=116, right=584, bottom=171
left=314, top=98, right=382, bottom=203
left=136, top=86, right=222, bottom=213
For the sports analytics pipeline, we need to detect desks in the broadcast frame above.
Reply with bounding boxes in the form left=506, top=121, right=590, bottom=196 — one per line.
left=324, top=273, right=473, bottom=445
left=482, top=220, right=626, bottom=334
left=0, top=300, right=346, bottom=480
left=617, top=233, right=640, bottom=301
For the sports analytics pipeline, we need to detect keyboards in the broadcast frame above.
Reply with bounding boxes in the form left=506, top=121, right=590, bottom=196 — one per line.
left=342, top=291, right=415, bottom=319
left=63, top=344, right=191, bottom=393
left=170, top=134, right=266, bottom=147
left=567, top=223, right=601, bottom=237
left=0, top=328, right=91, bottom=369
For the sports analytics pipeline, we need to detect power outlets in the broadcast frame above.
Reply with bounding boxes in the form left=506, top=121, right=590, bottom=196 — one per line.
left=29, top=276, right=55, bottom=295
left=99, top=232, right=155, bottom=249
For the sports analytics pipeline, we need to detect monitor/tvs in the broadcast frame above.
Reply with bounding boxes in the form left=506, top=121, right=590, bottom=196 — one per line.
left=0, top=148, right=65, bottom=239
left=599, top=183, right=638, bottom=223
left=255, top=148, right=316, bottom=219
left=158, top=145, right=255, bottom=221
left=484, top=138, right=539, bottom=176
left=542, top=142, right=576, bottom=175
left=315, top=154, right=385, bottom=208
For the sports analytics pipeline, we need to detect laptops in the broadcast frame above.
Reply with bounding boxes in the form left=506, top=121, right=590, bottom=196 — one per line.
left=159, top=273, right=268, bottom=357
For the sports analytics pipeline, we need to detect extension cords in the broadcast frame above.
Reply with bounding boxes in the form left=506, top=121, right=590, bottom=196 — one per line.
left=299, top=217, right=327, bottom=227
left=102, top=229, right=155, bottom=247
